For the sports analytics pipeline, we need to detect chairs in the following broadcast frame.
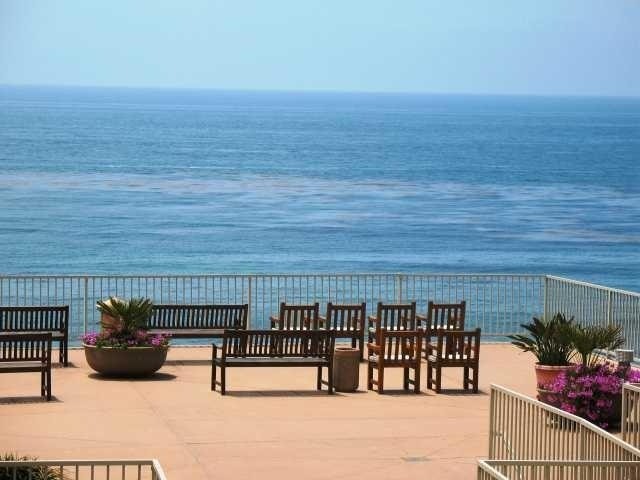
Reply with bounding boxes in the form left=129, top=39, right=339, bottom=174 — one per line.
left=369, top=301, right=416, bottom=357
left=319, top=303, right=367, bottom=361
left=428, top=328, right=481, bottom=394
left=417, top=301, right=466, bottom=355
left=269, top=302, right=318, bottom=357
left=367, top=327, right=423, bottom=394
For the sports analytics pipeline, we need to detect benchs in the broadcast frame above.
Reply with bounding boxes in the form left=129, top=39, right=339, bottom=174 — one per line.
left=211, top=329, right=335, bottom=396
left=0, top=306, right=68, bottom=368
left=0, top=333, right=52, bottom=402
left=146, top=304, right=248, bottom=359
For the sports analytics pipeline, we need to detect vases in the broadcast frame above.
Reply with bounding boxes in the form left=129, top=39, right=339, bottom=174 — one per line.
left=535, top=387, right=636, bottom=430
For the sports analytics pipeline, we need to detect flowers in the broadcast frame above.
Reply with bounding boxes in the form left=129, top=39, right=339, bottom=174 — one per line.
left=83, top=328, right=173, bottom=353
left=530, top=360, right=640, bottom=430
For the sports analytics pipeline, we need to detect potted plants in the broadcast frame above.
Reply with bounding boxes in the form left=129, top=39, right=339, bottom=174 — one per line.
left=505, top=313, right=577, bottom=387
left=82, top=294, right=171, bottom=378
